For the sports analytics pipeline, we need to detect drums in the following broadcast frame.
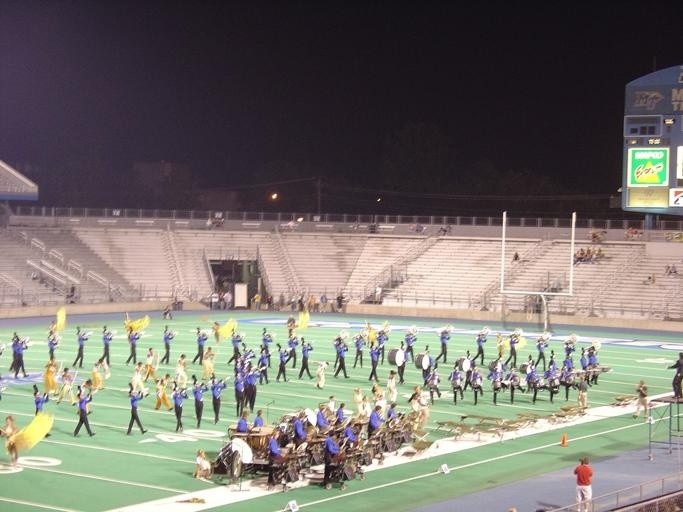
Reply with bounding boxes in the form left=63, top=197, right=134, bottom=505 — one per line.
left=518, top=362, right=532, bottom=374
left=488, top=360, right=502, bottom=372
left=387, top=348, right=406, bottom=367
left=414, top=353, right=431, bottom=370
left=219, top=438, right=254, bottom=471
left=457, top=356, right=471, bottom=373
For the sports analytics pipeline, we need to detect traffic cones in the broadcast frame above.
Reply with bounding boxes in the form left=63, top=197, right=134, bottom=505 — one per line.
left=561, top=433, right=567, bottom=447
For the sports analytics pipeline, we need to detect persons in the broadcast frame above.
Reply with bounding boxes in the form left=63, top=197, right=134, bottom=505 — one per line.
left=633, top=379, right=648, bottom=418
left=207, top=216, right=225, bottom=226
left=333, top=329, right=600, bottom=405
left=668, top=352, right=683, bottom=398
left=646, top=262, right=676, bottom=284
left=70, top=283, right=76, bottom=297
left=571, top=226, right=643, bottom=263
left=212, top=288, right=344, bottom=312
left=194, top=386, right=430, bottom=486
left=376, top=285, right=381, bottom=300
left=163, top=304, right=175, bottom=321
left=575, top=457, right=595, bottom=512
left=513, top=252, right=520, bottom=262
left=2, top=314, right=329, bottom=463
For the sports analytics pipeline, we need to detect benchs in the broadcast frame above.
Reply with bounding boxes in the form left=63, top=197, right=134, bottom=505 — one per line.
left=1, top=223, right=683, bottom=321
